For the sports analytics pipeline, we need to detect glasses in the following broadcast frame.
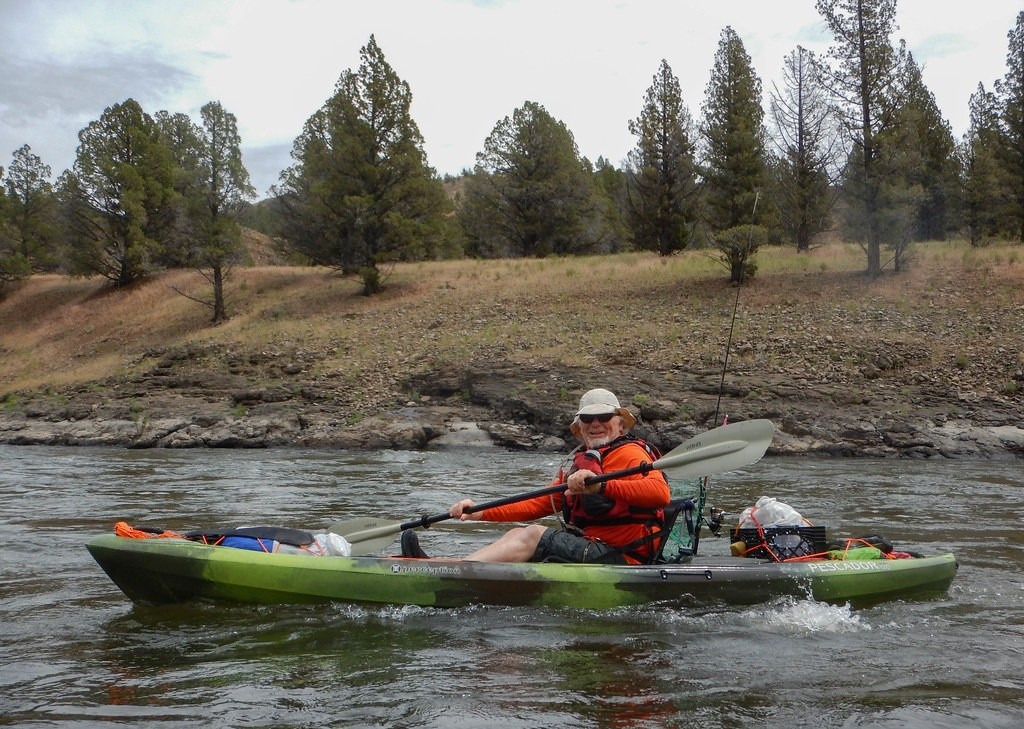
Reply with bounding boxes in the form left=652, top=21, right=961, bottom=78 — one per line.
left=579, top=413, right=620, bottom=423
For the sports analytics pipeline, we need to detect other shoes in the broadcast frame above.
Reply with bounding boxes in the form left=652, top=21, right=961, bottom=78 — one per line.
left=401, top=529, right=429, bottom=558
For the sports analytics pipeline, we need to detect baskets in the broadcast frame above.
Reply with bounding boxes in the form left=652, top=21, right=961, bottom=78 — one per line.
left=730, top=526, right=826, bottom=561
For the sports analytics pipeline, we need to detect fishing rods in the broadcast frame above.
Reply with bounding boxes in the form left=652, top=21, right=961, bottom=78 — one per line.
left=680, top=187, right=769, bottom=561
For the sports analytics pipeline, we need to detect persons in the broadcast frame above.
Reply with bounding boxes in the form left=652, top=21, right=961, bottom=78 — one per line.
left=449, top=389, right=670, bottom=563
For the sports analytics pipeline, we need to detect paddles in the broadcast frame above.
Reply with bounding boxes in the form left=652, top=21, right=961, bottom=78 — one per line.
left=321, top=416, right=782, bottom=561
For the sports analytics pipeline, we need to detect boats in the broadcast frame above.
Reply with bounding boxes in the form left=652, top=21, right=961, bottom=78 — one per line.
left=84, top=521, right=963, bottom=620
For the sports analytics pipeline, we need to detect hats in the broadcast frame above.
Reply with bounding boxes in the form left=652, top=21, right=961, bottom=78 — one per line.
left=569, top=388, right=637, bottom=444
